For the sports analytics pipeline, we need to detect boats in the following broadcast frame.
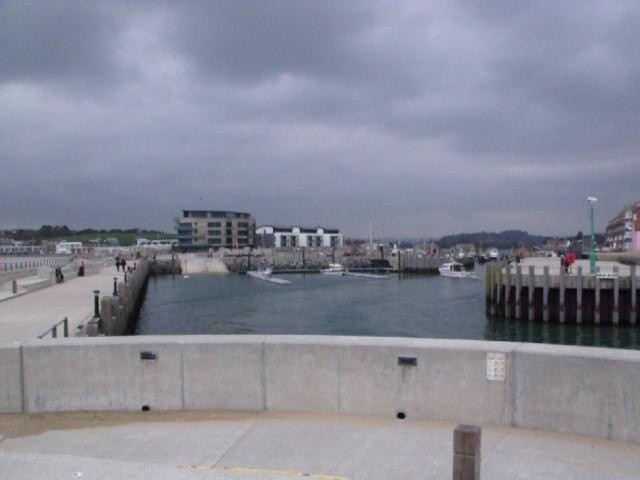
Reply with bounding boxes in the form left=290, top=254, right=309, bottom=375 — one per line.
left=320, top=263, right=348, bottom=276
left=245, top=265, right=290, bottom=286
left=475, top=246, right=512, bottom=262
left=439, top=261, right=469, bottom=278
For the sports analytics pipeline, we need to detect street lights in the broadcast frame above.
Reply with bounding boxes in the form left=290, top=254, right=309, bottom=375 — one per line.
left=586, top=196, right=598, bottom=273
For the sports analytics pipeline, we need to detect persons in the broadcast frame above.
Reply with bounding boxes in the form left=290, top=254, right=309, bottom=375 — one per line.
left=115, top=255, right=126, bottom=272
left=78, top=259, right=85, bottom=276
left=515, top=244, right=528, bottom=265
left=558, top=247, right=576, bottom=276
